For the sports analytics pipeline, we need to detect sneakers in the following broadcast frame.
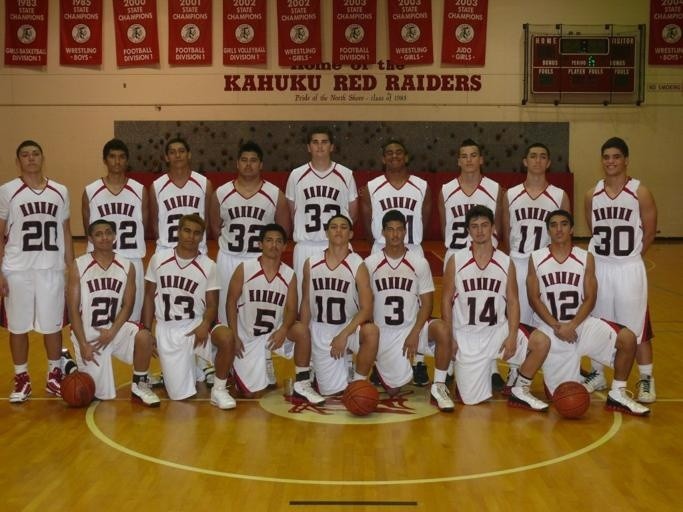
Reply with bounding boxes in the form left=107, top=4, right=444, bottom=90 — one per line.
left=149, top=372, right=163, bottom=385
left=9, top=372, right=32, bottom=403
left=369, top=360, right=656, bottom=416
left=45, top=367, right=65, bottom=397
left=293, top=371, right=325, bottom=406
left=210, top=386, right=236, bottom=410
left=266, top=358, right=276, bottom=386
left=61, top=348, right=78, bottom=380
left=131, top=375, right=160, bottom=407
left=203, top=365, right=215, bottom=388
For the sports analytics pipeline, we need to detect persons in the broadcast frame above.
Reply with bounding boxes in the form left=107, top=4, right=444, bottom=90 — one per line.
left=441, top=205, right=550, bottom=413
left=226, top=224, right=326, bottom=405
left=438, top=138, right=505, bottom=392
left=0, top=140, right=75, bottom=403
left=360, top=139, right=431, bottom=387
left=82, top=139, right=146, bottom=326
left=364, top=210, right=454, bottom=412
left=61, top=219, right=161, bottom=407
left=503, top=143, right=571, bottom=398
left=299, top=215, right=379, bottom=396
left=141, top=213, right=236, bottom=410
left=209, top=141, right=292, bottom=390
left=582, top=137, right=657, bottom=404
left=526, top=210, right=651, bottom=416
left=149, top=138, right=216, bottom=388
left=285, top=128, right=358, bottom=384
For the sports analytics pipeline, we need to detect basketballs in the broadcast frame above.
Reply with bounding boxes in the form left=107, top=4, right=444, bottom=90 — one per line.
left=60, top=371, right=95, bottom=407
left=553, top=382, right=592, bottom=419
left=343, top=380, right=379, bottom=416
